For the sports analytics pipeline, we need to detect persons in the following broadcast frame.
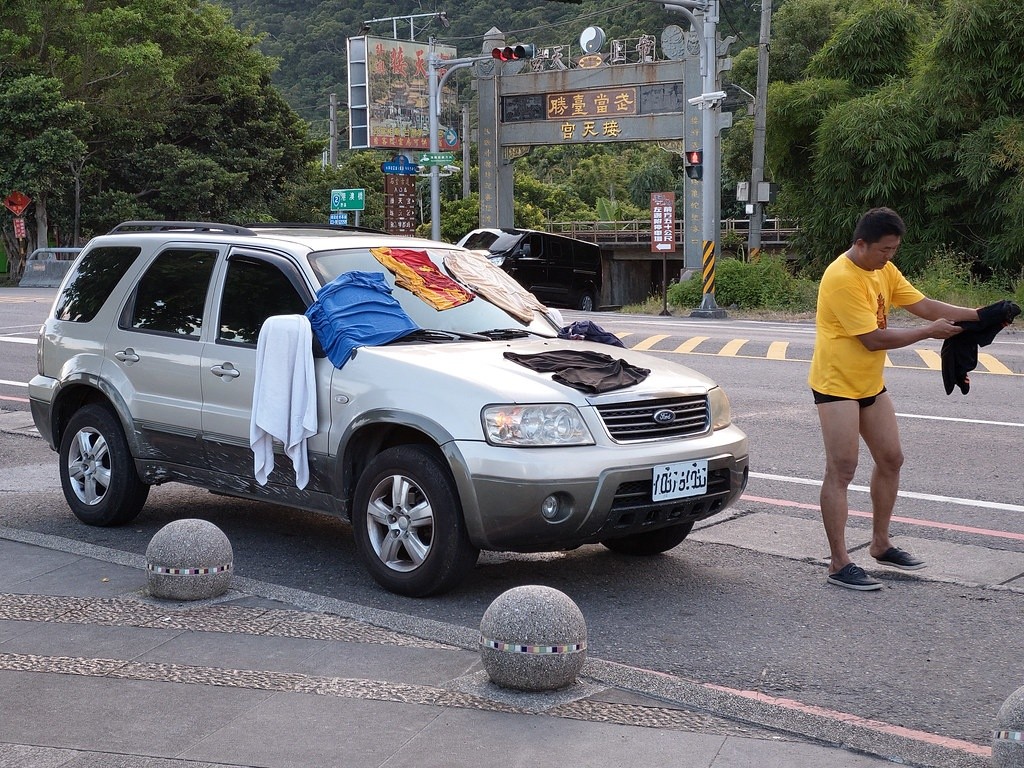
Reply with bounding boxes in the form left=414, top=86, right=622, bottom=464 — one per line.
left=807, top=207, right=1020, bottom=590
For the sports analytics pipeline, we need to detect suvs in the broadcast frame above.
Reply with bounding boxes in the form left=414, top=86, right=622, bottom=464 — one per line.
left=28, top=220, right=749, bottom=599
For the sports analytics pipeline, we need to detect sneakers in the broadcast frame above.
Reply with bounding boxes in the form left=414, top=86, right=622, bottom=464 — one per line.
left=876, top=546, right=927, bottom=570
left=827, top=563, right=883, bottom=590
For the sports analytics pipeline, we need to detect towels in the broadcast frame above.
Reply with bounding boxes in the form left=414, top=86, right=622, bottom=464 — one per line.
left=249, top=313, right=318, bottom=492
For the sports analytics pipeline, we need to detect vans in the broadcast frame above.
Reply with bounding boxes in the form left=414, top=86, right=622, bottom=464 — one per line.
left=453, top=226, right=604, bottom=313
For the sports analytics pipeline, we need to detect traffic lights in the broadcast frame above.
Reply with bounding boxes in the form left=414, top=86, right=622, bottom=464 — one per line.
left=491, top=43, right=537, bottom=62
left=685, top=166, right=701, bottom=180
left=685, top=151, right=701, bottom=164
